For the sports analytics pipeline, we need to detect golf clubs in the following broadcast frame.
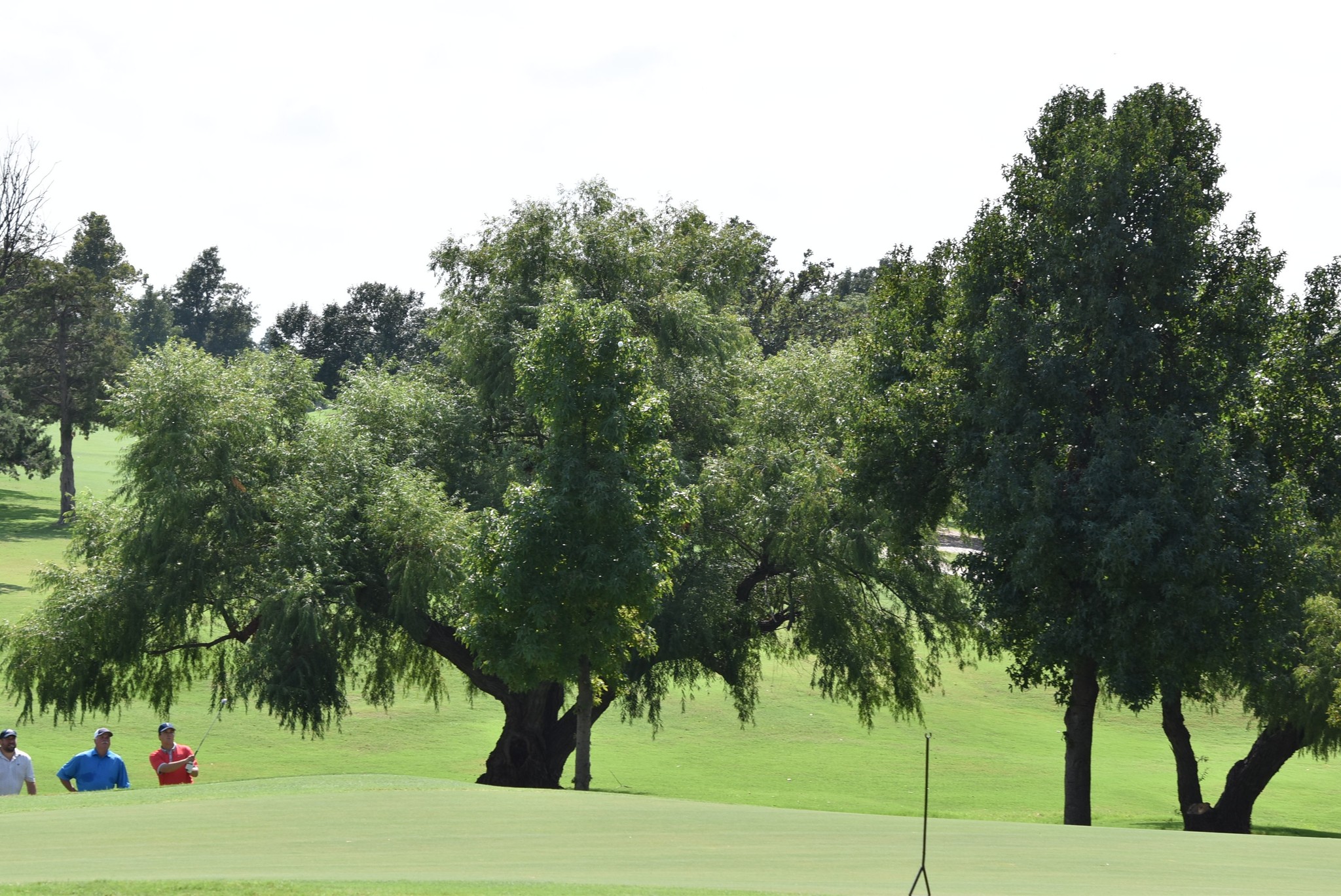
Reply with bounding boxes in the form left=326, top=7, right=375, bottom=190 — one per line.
left=185, top=698, right=228, bottom=772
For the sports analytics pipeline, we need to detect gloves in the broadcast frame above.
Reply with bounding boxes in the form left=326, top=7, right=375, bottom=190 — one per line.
left=185, top=761, right=193, bottom=773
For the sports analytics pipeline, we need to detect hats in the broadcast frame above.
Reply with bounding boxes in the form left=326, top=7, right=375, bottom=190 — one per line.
left=94, top=728, right=113, bottom=739
left=0, top=728, right=18, bottom=739
left=158, top=723, right=176, bottom=733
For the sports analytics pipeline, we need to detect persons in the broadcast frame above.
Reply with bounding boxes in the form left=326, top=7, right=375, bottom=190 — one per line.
left=0, top=729, right=37, bottom=796
left=149, top=723, right=199, bottom=786
left=56, top=728, right=130, bottom=792
